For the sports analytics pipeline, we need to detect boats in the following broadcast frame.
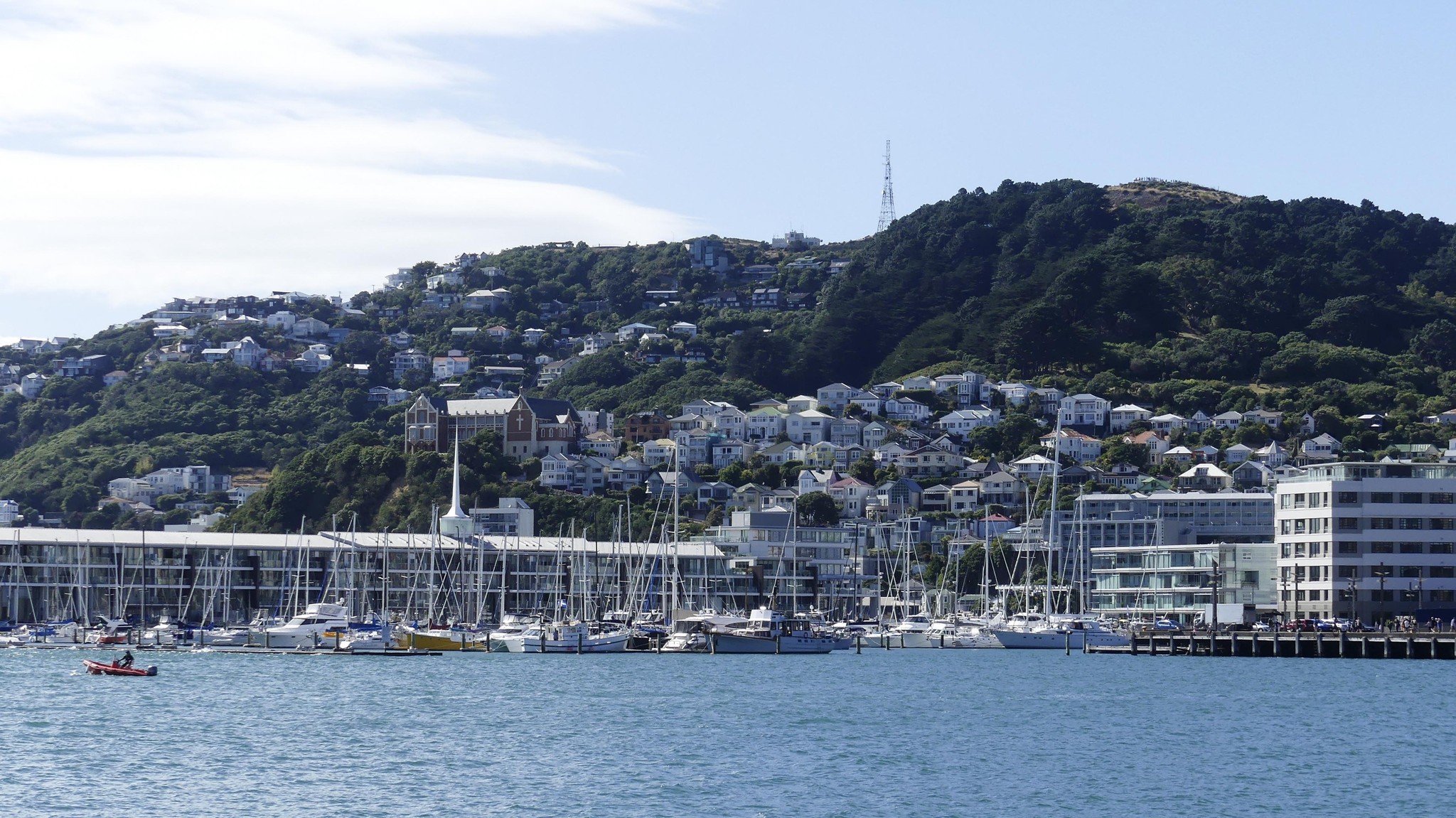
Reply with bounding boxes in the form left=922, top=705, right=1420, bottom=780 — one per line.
left=83, top=653, right=158, bottom=676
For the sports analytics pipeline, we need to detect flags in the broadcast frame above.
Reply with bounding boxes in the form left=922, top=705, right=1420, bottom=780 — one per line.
left=557, top=599, right=566, bottom=607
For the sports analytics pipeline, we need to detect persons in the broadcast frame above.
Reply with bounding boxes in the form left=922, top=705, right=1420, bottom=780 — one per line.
left=1400, top=616, right=1417, bottom=633
left=1449, top=617, right=1456, bottom=633
left=1207, top=624, right=1213, bottom=636
left=1298, top=617, right=1301, bottom=623
left=1389, top=621, right=1393, bottom=632
left=1287, top=620, right=1292, bottom=627
left=1268, top=617, right=1273, bottom=632
left=1374, top=622, right=1378, bottom=632
left=1394, top=617, right=1399, bottom=633
left=1347, top=619, right=1351, bottom=633
left=1313, top=617, right=1318, bottom=632
left=1379, top=625, right=1381, bottom=632
left=1355, top=617, right=1362, bottom=632
left=1332, top=614, right=1338, bottom=632
left=1426, top=617, right=1442, bottom=633
left=1274, top=619, right=1281, bottom=632
left=119, top=650, right=134, bottom=669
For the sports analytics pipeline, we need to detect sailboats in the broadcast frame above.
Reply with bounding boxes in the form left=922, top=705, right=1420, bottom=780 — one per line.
left=0, top=407, right=1210, bottom=658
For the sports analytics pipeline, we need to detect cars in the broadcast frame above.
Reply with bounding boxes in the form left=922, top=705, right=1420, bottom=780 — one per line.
left=1279, top=618, right=1375, bottom=632
left=1252, top=621, right=1271, bottom=632
left=1226, top=623, right=1252, bottom=636
left=1155, top=620, right=1186, bottom=631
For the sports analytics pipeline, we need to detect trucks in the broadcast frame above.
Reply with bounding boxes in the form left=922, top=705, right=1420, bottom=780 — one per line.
left=1193, top=603, right=1244, bottom=631
